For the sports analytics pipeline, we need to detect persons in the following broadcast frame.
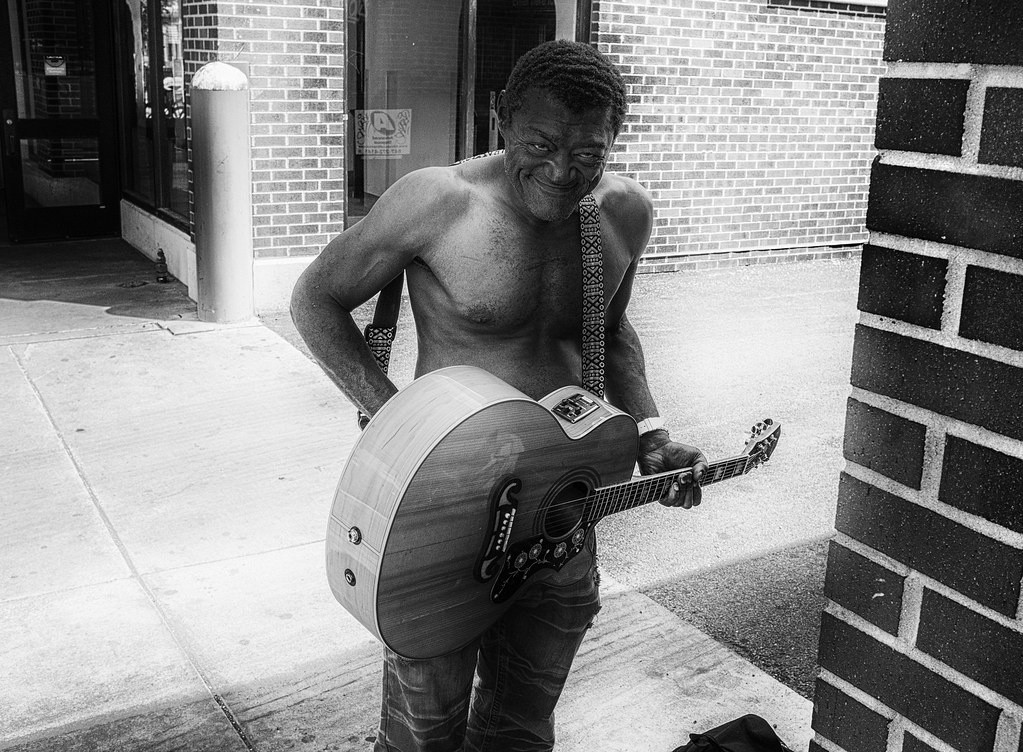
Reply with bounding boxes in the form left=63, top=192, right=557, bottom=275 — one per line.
left=284, top=36, right=708, bottom=752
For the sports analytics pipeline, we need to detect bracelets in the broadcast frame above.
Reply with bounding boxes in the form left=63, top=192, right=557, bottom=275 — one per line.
left=635, top=416, right=672, bottom=438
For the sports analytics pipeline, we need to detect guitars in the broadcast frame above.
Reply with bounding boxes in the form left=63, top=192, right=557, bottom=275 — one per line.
left=324, top=364, right=782, bottom=664
